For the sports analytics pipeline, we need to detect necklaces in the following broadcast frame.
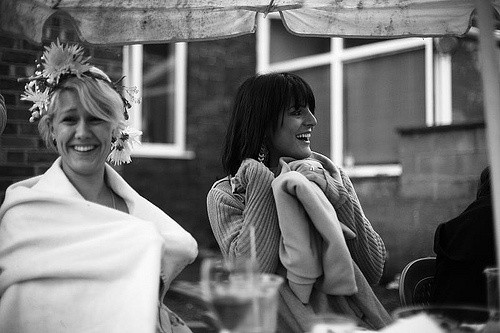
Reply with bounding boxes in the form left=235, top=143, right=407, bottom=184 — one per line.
left=109, top=187, right=118, bottom=210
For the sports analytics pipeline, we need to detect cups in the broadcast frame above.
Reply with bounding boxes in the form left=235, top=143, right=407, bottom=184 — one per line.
left=203, top=254, right=261, bottom=332
left=228, top=272, right=279, bottom=333
left=483, top=266, right=499, bottom=333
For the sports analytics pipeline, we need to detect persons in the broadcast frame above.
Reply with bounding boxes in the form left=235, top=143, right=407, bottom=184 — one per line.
left=208, top=72, right=396, bottom=333
left=1, top=38, right=199, bottom=333
left=430, top=160, right=499, bottom=324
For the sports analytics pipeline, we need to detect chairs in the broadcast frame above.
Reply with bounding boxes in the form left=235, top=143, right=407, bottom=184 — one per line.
left=399, top=254, right=441, bottom=321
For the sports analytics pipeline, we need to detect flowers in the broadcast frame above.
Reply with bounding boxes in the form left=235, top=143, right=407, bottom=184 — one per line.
left=14, top=36, right=146, bottom=168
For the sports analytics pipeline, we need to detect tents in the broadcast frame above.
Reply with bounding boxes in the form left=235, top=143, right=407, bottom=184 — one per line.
left=2, top=0, right=499, bottom=259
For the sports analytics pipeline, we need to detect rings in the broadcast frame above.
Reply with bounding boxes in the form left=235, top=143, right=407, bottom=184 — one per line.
left=310, top=165, right=315, bottom=170
